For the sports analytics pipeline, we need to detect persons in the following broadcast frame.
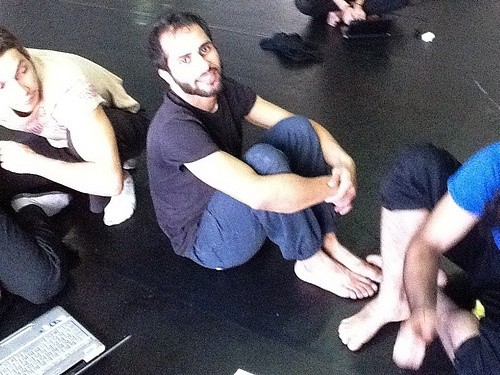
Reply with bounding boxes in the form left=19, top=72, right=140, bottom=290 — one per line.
left=0, top=191, right=70, bottom=304
left=338, top=139, right=500, bottom=375
left=0, top=26, right=147, bottom=227
left=146, top=10, right=385, bottom=300
left=294, top=0, right=410, bottom=26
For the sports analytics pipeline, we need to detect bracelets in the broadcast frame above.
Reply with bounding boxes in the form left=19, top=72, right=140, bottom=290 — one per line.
left=354, top=3, right=363, bottom=6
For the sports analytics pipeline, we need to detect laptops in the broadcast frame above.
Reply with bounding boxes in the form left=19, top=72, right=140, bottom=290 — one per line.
left=340, top=17, right=391, bottom=38
left=0, top=304, right=132, bottom=375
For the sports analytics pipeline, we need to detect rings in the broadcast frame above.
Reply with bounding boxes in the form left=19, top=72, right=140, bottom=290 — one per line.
left=330, top=18, right=332, bottom=20
